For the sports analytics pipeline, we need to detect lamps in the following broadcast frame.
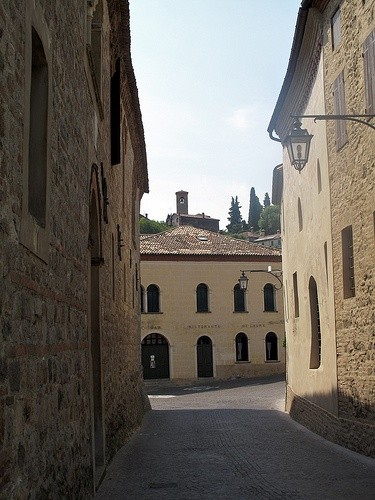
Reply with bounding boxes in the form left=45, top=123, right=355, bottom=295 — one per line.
left=238, top=269, right=283, bottom=293
left=282, top=112, right=375, bottom=175
